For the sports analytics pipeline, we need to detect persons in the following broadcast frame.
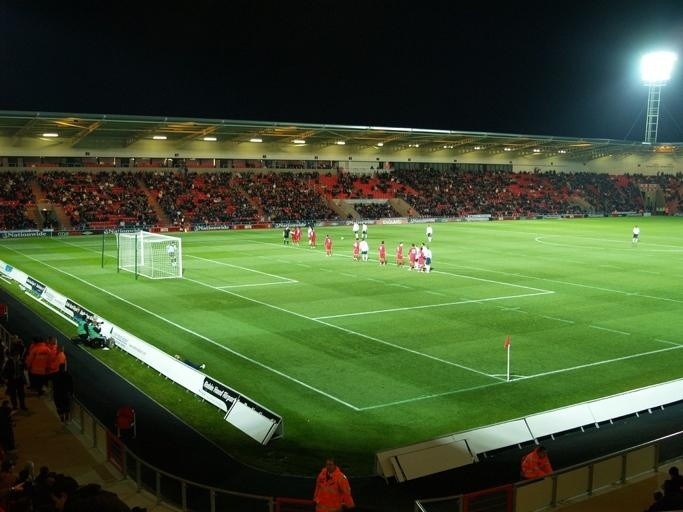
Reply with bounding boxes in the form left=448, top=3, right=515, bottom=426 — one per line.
left=649, top=466, right=683, bottom=512
left=3, top=335, right=72, bottom=429
left=308, top=225, right=316, bottom=246
left=395, top=241, right=432, bottom=273
left=0, top=456, right=130, bottom=512
left=175, top=354, right=206, bottom=370
left=632, top=225, right=640, bottom=243
left=283, top=225, right=301, bottom=245
left=427, top=225, right=433, bottom=242
left=324, top=236, right=332, bottom=256
left=313, top=459, right=355, bottom=511
left=521, top=443, right=553, bottom=480
left=379, top=241, right=386, bottom=265
left=117, top=401, right=133, bottom=444
left=77, top=315, right=110, bottom=351
left=353, top=221, right=368, bottom=261
left=0, top=162, right=683, bottom=231
left=0, top=397, right=17, bottom=454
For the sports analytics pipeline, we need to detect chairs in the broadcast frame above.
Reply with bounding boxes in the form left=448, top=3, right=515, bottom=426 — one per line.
left=1, top=156, right=683, bottom=233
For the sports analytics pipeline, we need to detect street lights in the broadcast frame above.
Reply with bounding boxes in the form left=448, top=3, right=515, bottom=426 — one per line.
left=640, top=48, right=675, bottom=141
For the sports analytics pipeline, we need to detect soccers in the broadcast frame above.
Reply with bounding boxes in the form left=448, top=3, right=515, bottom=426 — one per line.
left=341, top=237, right=344, bottom=239
